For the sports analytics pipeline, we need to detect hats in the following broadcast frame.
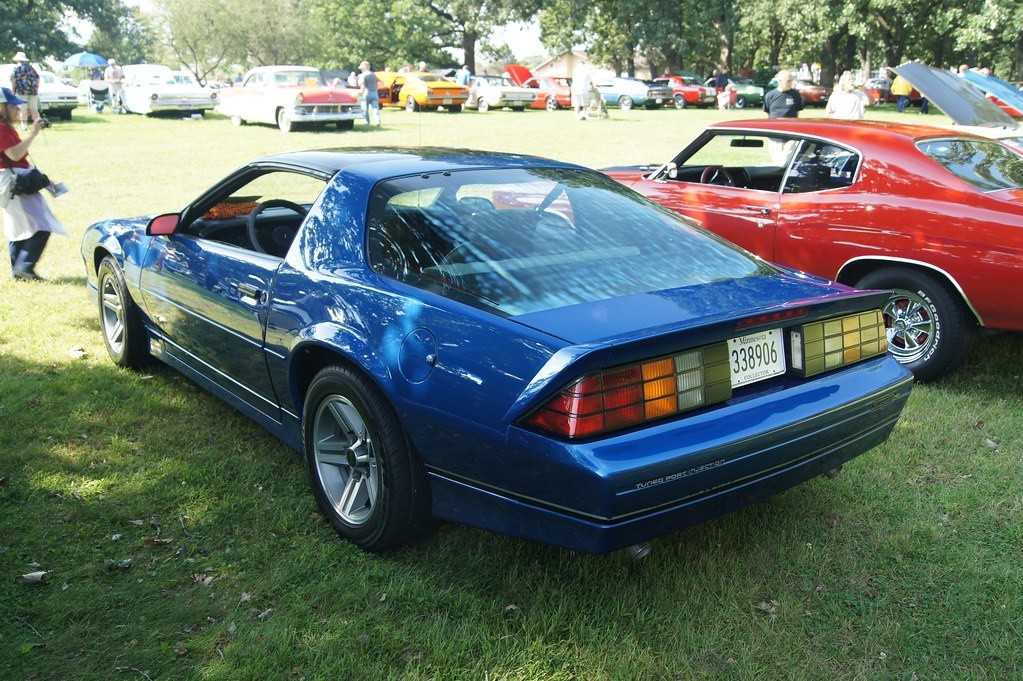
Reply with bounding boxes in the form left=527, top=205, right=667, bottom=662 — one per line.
left=12, top=52, right=29, bottom=61
left=0, top=87, right=27, bottom=104
left=358, top=61, right=370, bottom=70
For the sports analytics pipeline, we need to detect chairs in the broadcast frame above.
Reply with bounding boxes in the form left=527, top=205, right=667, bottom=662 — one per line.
left=438, top=209, right=540, bottom=264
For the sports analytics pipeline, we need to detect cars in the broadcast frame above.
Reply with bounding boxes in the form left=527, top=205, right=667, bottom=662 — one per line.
left=464, top=64, right=922, bottom=112
left=232, top=64, right=365, bottom=133
left=120, top=64, right=218, bottom=117
left=374, top=70, right=470, bottom=113
left=0, top=69, right=80, bottom=121
left=598, top=62, right=1023, bottom=385
left=79, top=145, right=915, bottom=555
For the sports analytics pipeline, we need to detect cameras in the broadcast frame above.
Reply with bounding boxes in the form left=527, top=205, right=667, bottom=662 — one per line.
left=40, top=118, right=51, bottom=128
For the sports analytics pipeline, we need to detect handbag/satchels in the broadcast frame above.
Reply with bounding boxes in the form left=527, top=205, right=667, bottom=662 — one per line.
left=10, top=170, right=50, bottom=200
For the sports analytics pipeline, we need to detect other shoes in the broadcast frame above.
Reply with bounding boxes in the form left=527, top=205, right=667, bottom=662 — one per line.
left=12, top=272, right=47, bottom=285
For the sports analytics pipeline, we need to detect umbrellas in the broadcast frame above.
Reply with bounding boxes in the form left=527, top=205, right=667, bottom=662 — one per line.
left=63, top=51, right=107, bottom=80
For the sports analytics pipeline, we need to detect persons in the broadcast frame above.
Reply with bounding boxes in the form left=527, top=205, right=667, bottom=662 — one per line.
left=88, top=59, right=126, bottom=115
left=0, top=87, right=67, bottom=281
left=374, top=61, right=428, bottom=72
left=348, top=72, right=357, bottom=87
left=798, top=62, right=820, bottom=83
left=454, top=65, right=471, bottom=112
left=216, top=73, right=243, bottom=87
left=661, top=66, right=670, bottom=78
left=875, top=69, right=929, bottom=115
left=704, top=68, right=729, bottom=108
left=571, top=60, right=591, bottom=120
left=825, top=71, right=869, bottom=120
left=502, top=72, right=512, bottom=109
left=950, top=64, right=989, bottom=125
left=332, top=78, right=345, bottom=88
left=762, top=69, right=803, bottom=118
left=358, top=61, right=381, bottom=126
left=10, top=51, right=50, bottom=130
